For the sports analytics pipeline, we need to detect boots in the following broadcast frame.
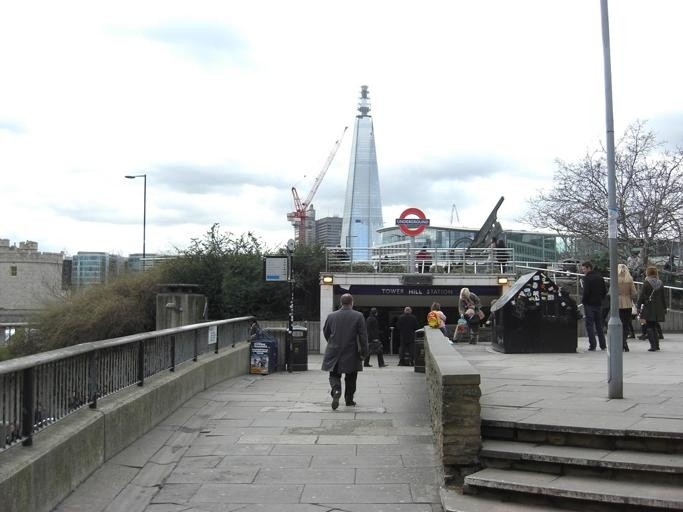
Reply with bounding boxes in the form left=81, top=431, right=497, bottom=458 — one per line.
left=620, top=326, right=665, bottom=351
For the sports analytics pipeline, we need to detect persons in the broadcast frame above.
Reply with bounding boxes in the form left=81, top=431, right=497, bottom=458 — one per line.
left=488, top=237, right=497, bottom=261
left=416, top=246, right=433, bottom=273
left=396, top=307, right=420, bottom=366
left=458, top=287, right=483, bottom=343
left=575, top=260, right=668, bottom=352
left=364, top=307, right=389, bottom=368
left=431, top=302, right=454, bottom=345
left=497, top=240, right=508, bottom=273
left=320, top=293, right=369, bottom=410
left=332, top=244, right=350, bottom=261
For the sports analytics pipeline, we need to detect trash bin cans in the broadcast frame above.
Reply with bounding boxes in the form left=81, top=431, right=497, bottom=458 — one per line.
left=414, top=329, right=425, bottom=373
left=563, top=259, right=577, bottom=278
left=261, top=326, right=308, bottom=375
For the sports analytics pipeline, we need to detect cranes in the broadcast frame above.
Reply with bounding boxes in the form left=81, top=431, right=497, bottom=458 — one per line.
left=286, top=125, right=348, bottom=248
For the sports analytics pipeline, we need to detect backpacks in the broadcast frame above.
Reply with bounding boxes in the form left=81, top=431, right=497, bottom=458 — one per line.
left=427, top=310, right=441, bottom=328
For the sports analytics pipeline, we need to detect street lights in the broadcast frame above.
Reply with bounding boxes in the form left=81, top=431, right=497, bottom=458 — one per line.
left=125, top=173, right=147, bottom=271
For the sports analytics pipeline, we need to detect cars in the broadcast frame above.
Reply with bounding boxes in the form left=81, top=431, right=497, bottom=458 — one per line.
left=559, top=259, right=580, bottom=276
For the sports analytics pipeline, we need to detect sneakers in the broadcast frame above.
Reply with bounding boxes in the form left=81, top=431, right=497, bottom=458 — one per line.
left=346, top=402, right=357, bottom=407
left=379, top=364, right=388, bottom=367
left=331, top=391, right=341, bottom=409
left=587, top=344, right=606, bottom=351
left=364, top=364, right=373, bottom=368
